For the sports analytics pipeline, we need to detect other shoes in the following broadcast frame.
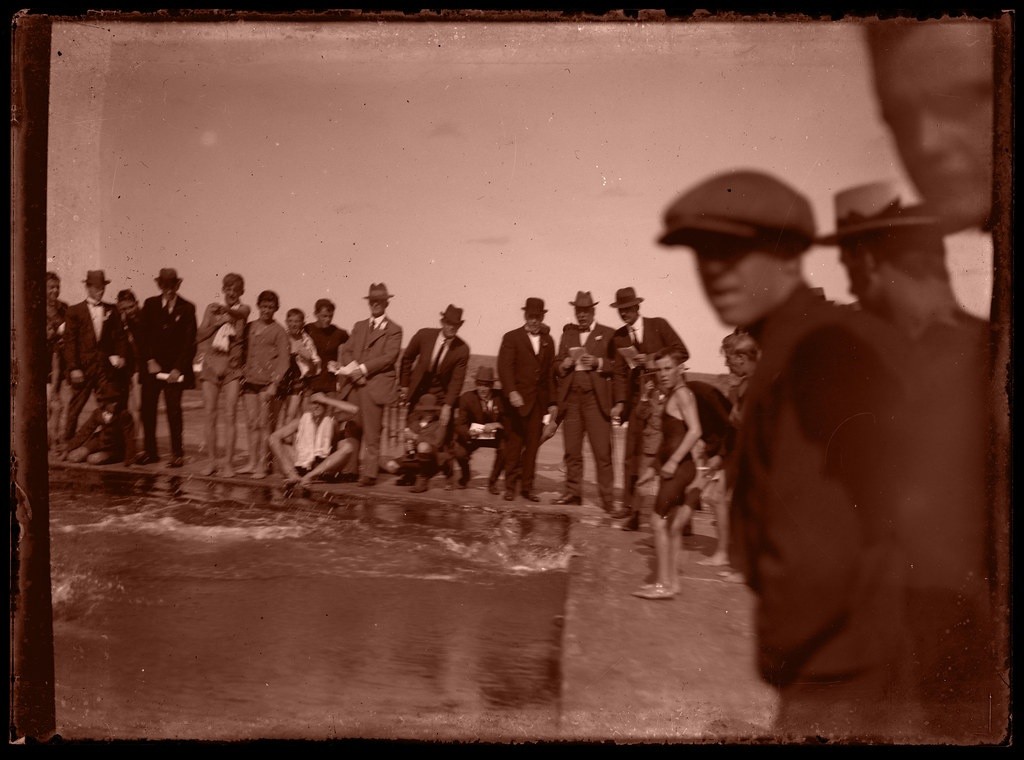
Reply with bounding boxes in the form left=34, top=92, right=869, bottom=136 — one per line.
left=134, top=453, right=273, bottom=479
left=631, top=555, right=747, bottom=599
left=336, top=474, right=639, bottom=532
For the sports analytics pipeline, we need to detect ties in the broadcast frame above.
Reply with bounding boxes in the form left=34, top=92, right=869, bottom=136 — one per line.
left=161, top=301, right=169, bottom=316
left=369, top=322, right=376, bottom=332
left=424, top=339, right=446, bottom=389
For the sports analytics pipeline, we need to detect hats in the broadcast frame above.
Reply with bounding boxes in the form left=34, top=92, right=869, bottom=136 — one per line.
left=520, top=298, right=548, bottom=314
left=610, top=287, right=644, bottom=308
left=440, top=303, right=466, bottom=326
left=656, top=168, right=817, bottom=247
left=362, top=283, right=394, bottom=300
left=82, top=270, right=111, bottom=286
left=154, top=268, right=182, bottom=287
left=471, top=366, right=499, bottom=382
left=568, top=291, right=598, bottom=309
left=95, top=383, right=122, bottom=401
left=413, top=394, right=441, bottom=411
left=819, top=181, right=940, bottom=245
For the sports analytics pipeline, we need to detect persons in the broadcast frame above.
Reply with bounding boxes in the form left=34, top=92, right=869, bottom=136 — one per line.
left=867, top=20, right=994, bottom=230
left=553, top=291, right=616, bottom=513
left=453, top=366, right=504, bottom=495
left=622, top=349, right=703, bottom=597
left=47, top=271, right=140, bottom=467
left=387, top=305, right=470, bottom=493
left=235, top=290, right=290, bottom=479
left=686, top=381, right=736, bottom=565
left=301, top=299, right=349, bottom=413
left=275, top=308, right=322, bottom=431
left=342, top=283, right=402, bottom=487
left=659, top=172, right=1009, bottom=737
left=720, top=333, right=756, bottom=582
left=136, top=268, right=197, bottom=467
left=610, top=287, right=691, bottom=519
left=196, top=273, right=251, bottom=477
left=497, top=298, right=558, bottom=502
left=268, top=388, right=359, bottom=489
